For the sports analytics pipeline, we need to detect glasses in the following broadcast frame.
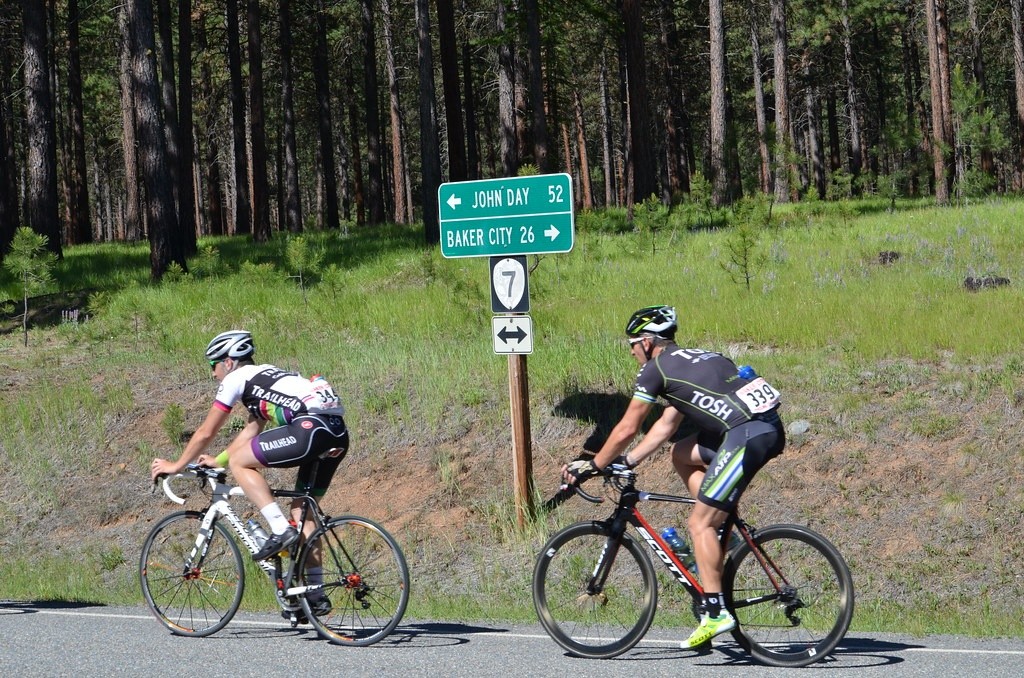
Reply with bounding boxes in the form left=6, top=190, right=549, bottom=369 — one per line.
left=210, top=358, right=227, bottom=371
left=628, top=334, right=667, bottom=349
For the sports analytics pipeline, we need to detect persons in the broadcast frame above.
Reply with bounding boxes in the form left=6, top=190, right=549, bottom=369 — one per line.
left=560, top=304, right=786, bottom=649
left=151, top=330, right=349, bottom=620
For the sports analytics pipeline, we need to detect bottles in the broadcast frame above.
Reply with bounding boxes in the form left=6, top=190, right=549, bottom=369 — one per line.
left=309, top=373, right=324, bottom=383
left=738, top=365, right=757, bottom=382
left=277, top=520, right=296, bottom=557
left=661, top=527, right=696, bottom=573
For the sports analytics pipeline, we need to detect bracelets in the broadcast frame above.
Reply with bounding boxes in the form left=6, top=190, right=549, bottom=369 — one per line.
left=626, top=452, right=637, bottom=465
left=216, top=450, right=230, bottom=468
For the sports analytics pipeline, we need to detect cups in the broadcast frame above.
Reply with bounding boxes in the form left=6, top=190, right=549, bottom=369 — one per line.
left=246, top=518, right=270, bottom=549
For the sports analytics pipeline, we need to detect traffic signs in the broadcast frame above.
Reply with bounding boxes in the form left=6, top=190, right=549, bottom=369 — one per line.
left=437, top=170, right=575, bottom=220
left=438, top=211, right=575, bottom=259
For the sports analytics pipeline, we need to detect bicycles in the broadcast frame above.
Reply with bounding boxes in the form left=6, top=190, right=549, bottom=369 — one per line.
left=531, top=450, right=856, bottom=668
left=137, top=447, right=410, bottom=647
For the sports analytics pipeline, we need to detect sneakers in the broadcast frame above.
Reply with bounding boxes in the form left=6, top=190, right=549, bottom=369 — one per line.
left=251, top=526, right=299, bottom=561
left=717, top=530, right=740, bottom=548
left=680, top=608, right=736, bottom=648
left=282, top=594, right=332, bottom=619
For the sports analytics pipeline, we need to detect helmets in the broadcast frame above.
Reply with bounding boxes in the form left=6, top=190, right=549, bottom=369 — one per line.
left=205, top=330, right=254, bottom=360
left=626, top=305, right=677, bottom=339
